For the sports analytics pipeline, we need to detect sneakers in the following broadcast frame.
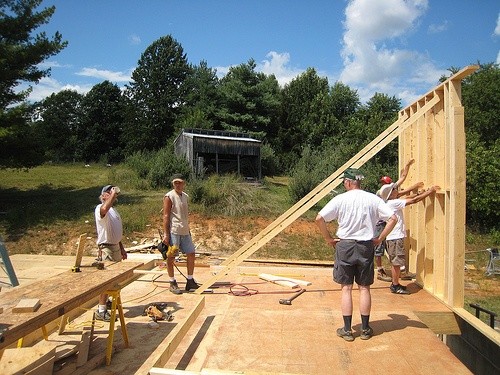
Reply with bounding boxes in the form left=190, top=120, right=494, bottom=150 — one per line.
left=399, top=268, right=416, bottom=279
left=360, top=326, right=373, bottom=340
left=390, top=282, right=411, bottom=294
left=377, top=268, right=392, bottom=282
left=336, top=327, right=355, bottom=342
left=95, top=309, right=117, bottom=322
left=186, top=278, right=201, bottom=291
left=106, top=301, right=118, bottom=309
left=169, top=280, right=182, bottom=294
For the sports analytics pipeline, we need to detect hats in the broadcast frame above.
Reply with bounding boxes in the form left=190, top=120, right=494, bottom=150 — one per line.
left=377, top=176, right=392, bottom=184
left=376, top=182, right=395, bottom=202
left=172, top=173, right=186, bottom=182
left=101, top=185, right=115, bottom=192
left=339, top=167, right=365, bottom=181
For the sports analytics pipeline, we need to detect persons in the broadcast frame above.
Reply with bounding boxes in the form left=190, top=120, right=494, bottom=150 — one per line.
left=95, top=185, right=128, bottom=321
left=373, top=158, right=418, bottom=281
left=316, top=168, right=398, bottom=340
left=381, top=182, right=441, bottom=294
left=161, top=174, right=201, bottom=293
